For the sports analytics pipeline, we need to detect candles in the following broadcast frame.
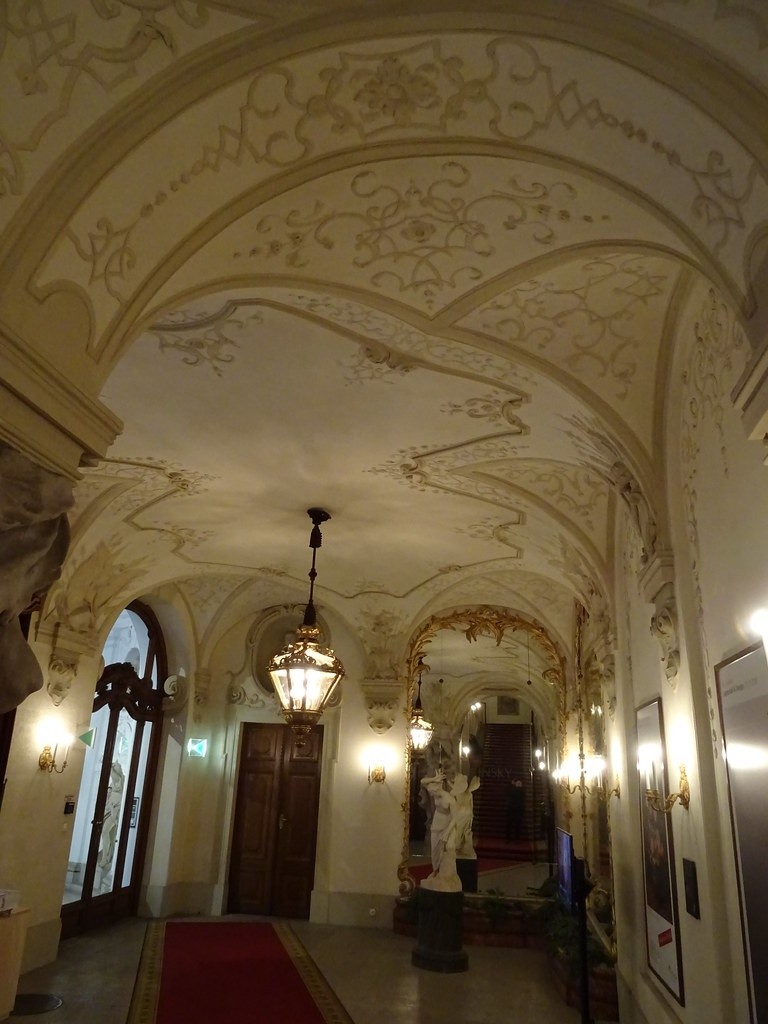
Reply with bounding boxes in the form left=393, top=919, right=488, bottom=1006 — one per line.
left=64, top=746, right=70, bottom=761
left=598, top=773, right=602, bottom=787
left=52, top=744, right=58, bottom=761
left=653, top=762, right=658, bottom=790
left=646, top=773, right=650, bottom=789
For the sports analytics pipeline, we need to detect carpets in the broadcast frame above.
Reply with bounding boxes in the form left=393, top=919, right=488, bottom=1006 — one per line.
left=126, top=921, right=355, bottom=1024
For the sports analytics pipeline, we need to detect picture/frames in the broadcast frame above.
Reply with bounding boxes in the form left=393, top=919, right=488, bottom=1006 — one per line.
left=633, top=697, right=685, bottom=1008
left=714, top=639, right=768, bottom=1024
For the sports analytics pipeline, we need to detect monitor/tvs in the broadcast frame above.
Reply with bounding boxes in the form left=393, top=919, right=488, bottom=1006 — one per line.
left=554, top=827, right=578, bottom=917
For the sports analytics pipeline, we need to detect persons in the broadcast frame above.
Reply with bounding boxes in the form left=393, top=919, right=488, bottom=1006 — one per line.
left=98, top=762, right=125, bottom=867
left=421, top=769, right=461, bottom=880
left=447, top=774, right=475, bottom=855
left=504, top=778, right=525, bottom=843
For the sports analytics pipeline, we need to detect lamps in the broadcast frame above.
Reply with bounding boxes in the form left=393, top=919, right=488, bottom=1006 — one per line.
left=265, top=507, right=345, bottom=747
left=410, top=655, right=434, bottom=760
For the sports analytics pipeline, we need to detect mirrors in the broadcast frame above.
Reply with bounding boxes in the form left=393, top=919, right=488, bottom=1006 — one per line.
left=397, top=607, right=567, bottom=913
